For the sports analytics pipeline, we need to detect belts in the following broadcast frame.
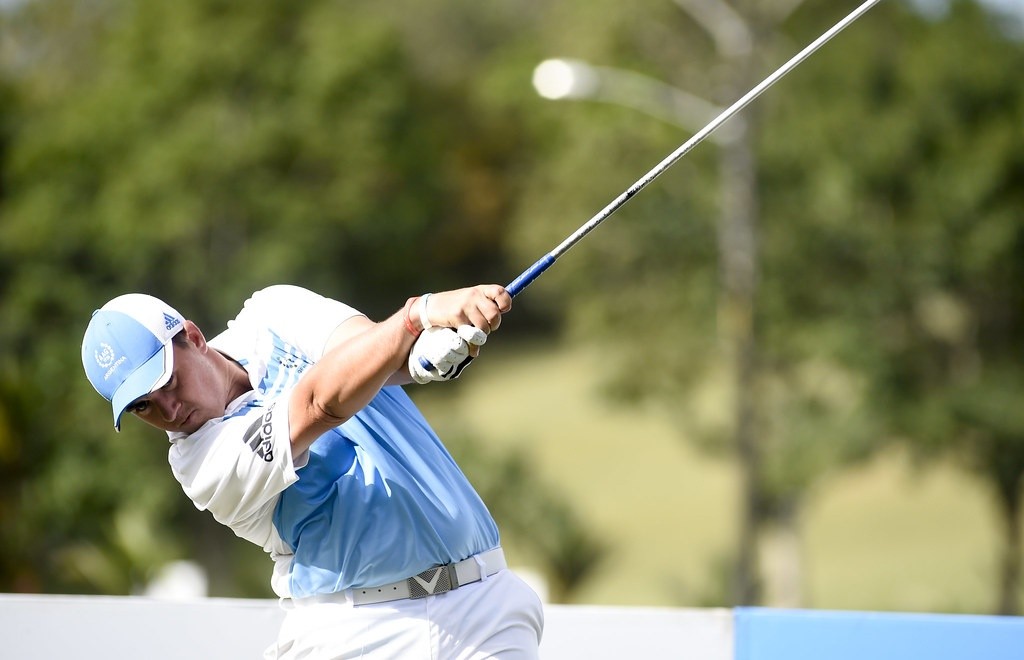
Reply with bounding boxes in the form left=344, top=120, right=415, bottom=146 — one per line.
left=290, top=544, right=506, bottom=606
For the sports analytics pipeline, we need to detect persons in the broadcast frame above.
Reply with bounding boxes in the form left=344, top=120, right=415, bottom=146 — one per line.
left=81, top=283, right=546, bottom=660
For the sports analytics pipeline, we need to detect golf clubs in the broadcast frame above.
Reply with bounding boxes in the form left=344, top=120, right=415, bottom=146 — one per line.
left=418, top=0, right=881, bottom=370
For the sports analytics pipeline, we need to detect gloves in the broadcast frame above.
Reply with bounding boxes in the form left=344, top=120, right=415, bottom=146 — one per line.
left=408, top=324, right=488, bottom=385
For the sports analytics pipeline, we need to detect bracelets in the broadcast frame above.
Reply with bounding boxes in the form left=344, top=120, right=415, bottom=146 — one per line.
left=402, top=296, right=419, bottom=338
left=419, top=292, right=433, bottom=330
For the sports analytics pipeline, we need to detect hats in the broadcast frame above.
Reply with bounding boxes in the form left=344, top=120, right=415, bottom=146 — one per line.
left=81, top=293, right=189, bottom=433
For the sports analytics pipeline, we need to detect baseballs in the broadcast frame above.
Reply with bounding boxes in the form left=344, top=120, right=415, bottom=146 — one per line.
left=528, top=58, right=572, bottom=101
